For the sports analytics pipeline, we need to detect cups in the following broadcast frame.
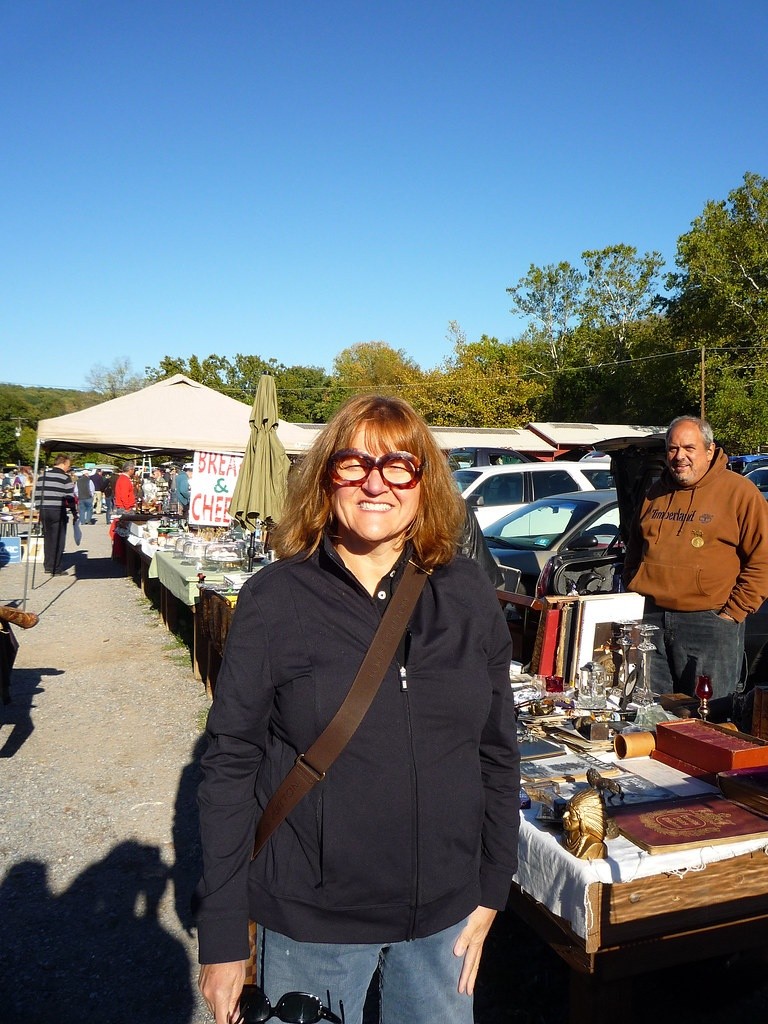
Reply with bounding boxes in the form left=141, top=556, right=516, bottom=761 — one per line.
left=533, top=674, right=547, bottom=692
left=546, top=676, right=563, bottom=692
left=144, top=508, right=150, bottom=514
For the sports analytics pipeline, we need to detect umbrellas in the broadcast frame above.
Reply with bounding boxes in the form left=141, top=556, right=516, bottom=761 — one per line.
left=227, top=370, right=291, bottom=571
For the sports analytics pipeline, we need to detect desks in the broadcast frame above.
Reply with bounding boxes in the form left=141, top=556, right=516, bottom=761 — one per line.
left=0, top=515, right=40, bottom=537
left=507, top=672, right=768, bottom=1024
left=110, top=510, right=281, bottom=699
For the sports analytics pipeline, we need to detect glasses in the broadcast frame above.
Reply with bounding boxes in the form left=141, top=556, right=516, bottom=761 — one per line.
left=233, top=984, right=345, bottom=1024
left=325, top=448, right=423, bottom=490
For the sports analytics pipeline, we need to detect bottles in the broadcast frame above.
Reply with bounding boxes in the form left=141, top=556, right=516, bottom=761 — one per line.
left=158, top=519, right=177, bottom=547
left=136, top=498, right=142, bottom=515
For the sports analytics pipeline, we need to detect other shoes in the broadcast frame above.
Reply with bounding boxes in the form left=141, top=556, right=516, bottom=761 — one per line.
left=107, top=522, right=111, bottom=525
left=44, top=569, right=68, bottom=576
left=80, top=520, right=93, bottom=525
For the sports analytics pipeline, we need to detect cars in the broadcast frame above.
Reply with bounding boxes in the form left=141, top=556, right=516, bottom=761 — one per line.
left=449, top=430, right=768, bottom=689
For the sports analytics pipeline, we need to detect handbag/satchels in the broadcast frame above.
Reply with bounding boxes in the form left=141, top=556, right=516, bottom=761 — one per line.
left=73, top=518, right=82, bottom=546
left=244, top=918, right=258, bottom=984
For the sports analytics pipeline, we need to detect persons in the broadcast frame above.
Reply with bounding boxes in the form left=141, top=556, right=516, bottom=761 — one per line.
left=620, top=416, right=768, bottom=723
left=561, top=787, right=609, bottom=859
left=68, top=460, right=191, bottom=525
left=0, top=471, right=33, bottom=491
left=184, top=393, right=521, bottom=1024
left=35, top=453, right=78, bottom=577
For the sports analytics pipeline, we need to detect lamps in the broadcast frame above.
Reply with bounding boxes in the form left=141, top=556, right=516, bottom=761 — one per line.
left=696, top=675, right=712, bottom=722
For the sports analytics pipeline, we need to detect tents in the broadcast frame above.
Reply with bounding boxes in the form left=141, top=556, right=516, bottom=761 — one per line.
left=22, top=373, right=316, bottom=614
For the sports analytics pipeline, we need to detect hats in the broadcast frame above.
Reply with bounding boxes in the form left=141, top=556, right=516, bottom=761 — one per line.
left=82, top=470, right=88, bottom=474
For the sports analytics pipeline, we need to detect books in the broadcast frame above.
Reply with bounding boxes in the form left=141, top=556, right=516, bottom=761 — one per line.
left=529, top=591, right=645, bottom=687
left=516, top=737, right=768, bottom=856
